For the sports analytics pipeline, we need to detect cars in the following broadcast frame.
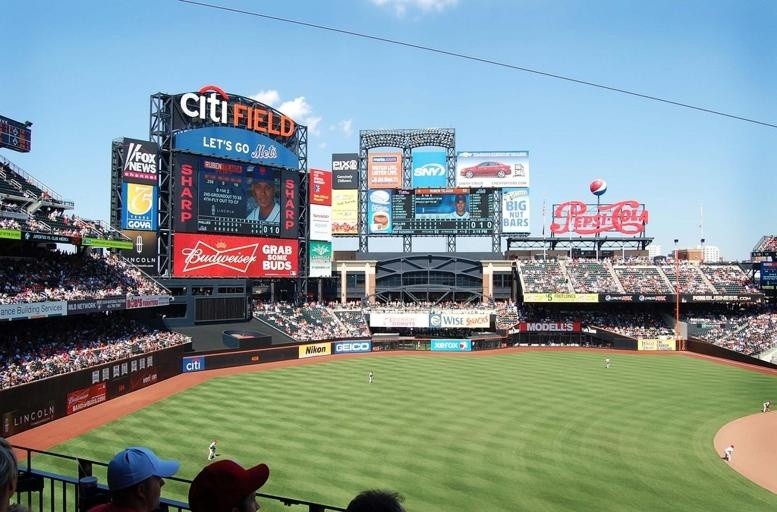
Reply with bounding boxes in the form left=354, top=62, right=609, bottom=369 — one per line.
left=460, top=161, right=511, bottom=179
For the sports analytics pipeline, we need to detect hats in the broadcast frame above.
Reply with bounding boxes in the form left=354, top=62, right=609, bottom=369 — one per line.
left=107, top=445, right=179, bottom=491
left=251, top=166, right=274, bottom=185
left=455, top=194, right=466, bottom=202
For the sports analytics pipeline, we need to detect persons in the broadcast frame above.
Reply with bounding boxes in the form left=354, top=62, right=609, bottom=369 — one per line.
left=88, top=445, right=179, bottom=511
left=345, top=487, right=407, bottom=511
left=1, top=190, right=113, bottom=240
left=723, top=444, right=734, bottom=461
left=206, top=439, right=216, bottom=460
left=1, top=310, right=192, bottom=390
left=251, top=292, right=520, bottom=343
left=368, top=370, right=373, bottom=383
left=762, top=399, right=771, bottom=412
left=0, top=436, right=26, bottom=511
left=513, top=233, right=777, bottom=357
left=449, top=194, right=471, bottom=221
left=605, top=357, right=611, bottom=368
left=245, top=167, right=281, bottom=223
left=1, top=246, right=168, bottom=304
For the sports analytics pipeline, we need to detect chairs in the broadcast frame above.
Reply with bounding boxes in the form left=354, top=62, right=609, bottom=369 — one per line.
left=255, top=261, right=777, bottom=355
left=0, top=162, right=121, bottom=241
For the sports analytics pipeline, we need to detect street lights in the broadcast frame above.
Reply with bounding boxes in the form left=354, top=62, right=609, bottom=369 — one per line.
left=674, top=239, right=679, bottom=259
left=701, top=239, right=705, bottom=262
